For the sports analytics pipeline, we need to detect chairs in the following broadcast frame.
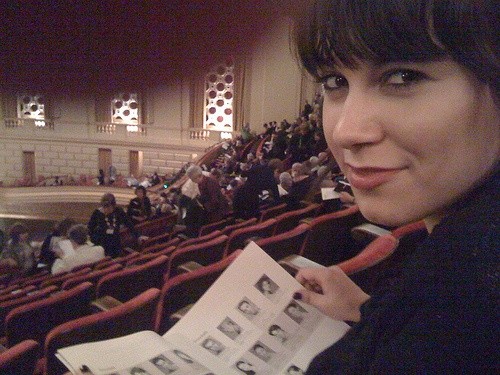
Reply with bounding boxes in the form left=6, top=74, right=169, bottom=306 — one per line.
left=0, top=201, right=431, bottom=375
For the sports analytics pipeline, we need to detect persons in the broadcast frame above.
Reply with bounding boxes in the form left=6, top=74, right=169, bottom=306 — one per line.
left=292, top=0, right=500, bottom=375
left=127, top=161, right=190, bottom=225
left=257, top=90, right=357, bottom=214
left=179, top=121, right=279, bottom=228
left=54, top=165, right=118, bottom=186
left=130, top=278, right=309, bottom=375
left=0, top=193, right=149, bottom=282
